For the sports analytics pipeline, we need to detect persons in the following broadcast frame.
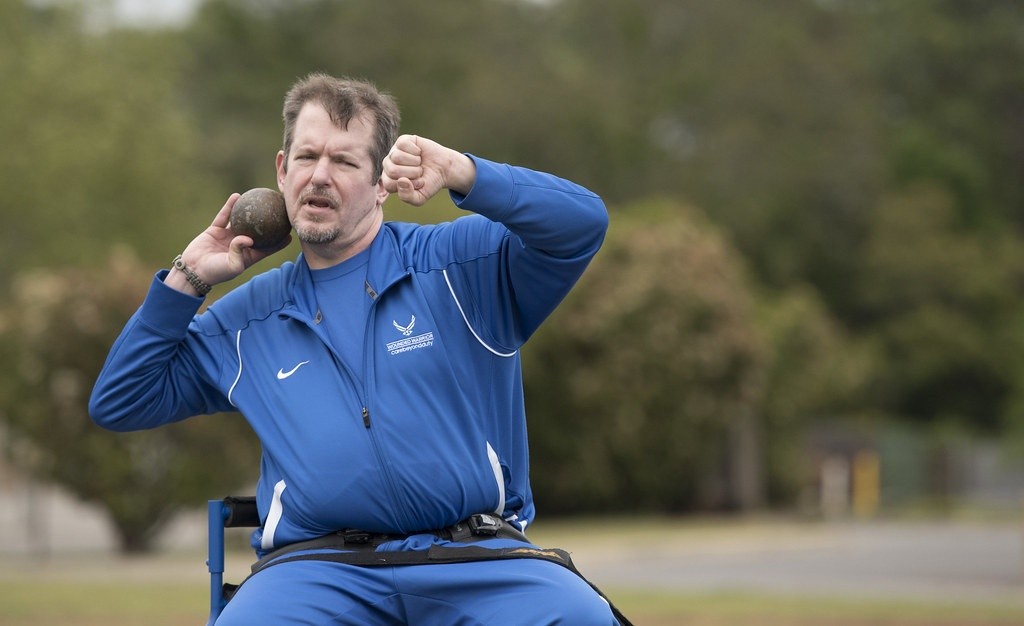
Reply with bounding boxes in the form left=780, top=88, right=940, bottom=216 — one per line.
left=89, top=74, right=629, bottom=626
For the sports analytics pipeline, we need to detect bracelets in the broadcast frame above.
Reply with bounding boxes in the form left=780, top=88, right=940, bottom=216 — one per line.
left=172, top=253, right=212, bottom=296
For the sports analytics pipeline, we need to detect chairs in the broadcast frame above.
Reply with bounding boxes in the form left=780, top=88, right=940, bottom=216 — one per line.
left=205, top=493, right=259, bottom=625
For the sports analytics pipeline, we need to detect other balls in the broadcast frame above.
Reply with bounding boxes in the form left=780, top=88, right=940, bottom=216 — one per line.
left=229, top=186, right=292, bottom=254
